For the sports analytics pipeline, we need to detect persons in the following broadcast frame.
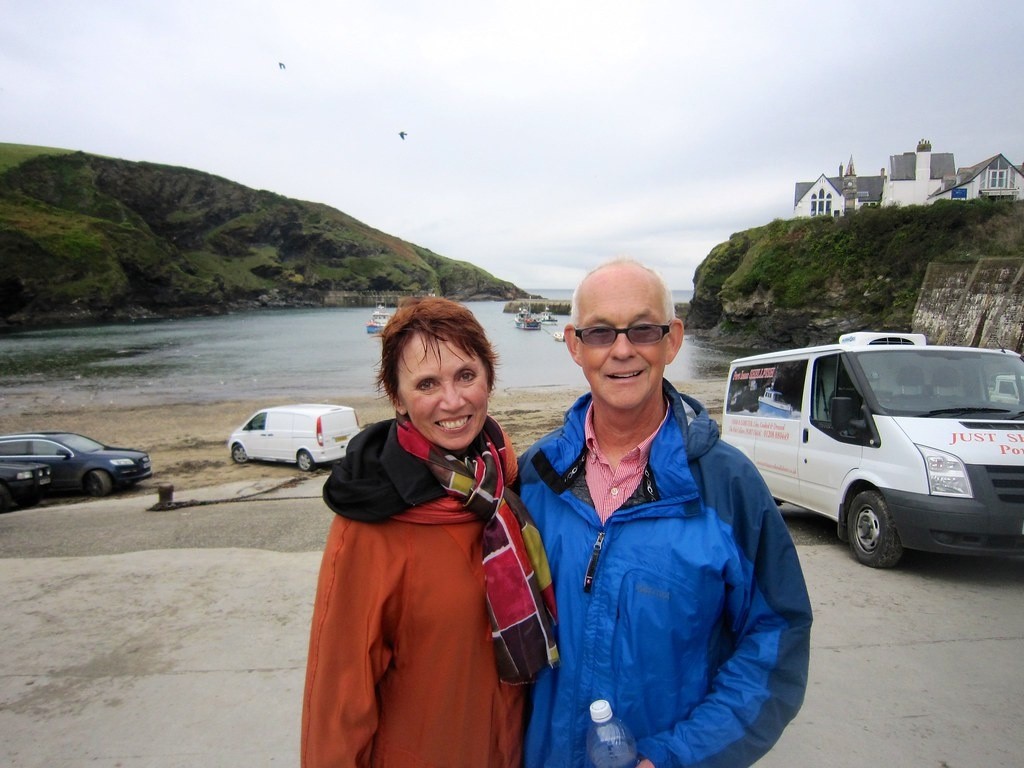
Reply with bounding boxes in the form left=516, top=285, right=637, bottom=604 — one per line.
left=299, top=297, right=529, bottom=768
left=512, top=258, right=812, bottom=768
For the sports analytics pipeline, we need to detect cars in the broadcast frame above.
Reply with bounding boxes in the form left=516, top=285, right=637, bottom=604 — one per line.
left=0, top=461, right=52, bottom=513
left=0, top=431, right=153, bottom=497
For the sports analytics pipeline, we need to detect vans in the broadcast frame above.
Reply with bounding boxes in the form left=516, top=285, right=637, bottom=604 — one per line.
left=228, top=404, right=361, bottom=472
left=721, top=332, right=1024, bottom=569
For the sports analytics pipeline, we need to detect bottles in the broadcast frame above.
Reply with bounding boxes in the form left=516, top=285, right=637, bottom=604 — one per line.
left=585, top=700, right=639, bottom=768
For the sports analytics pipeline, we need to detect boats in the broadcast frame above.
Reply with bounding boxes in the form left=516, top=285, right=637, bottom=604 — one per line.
left=758, top=389, right=793, bottom=419
left=366, top=305, right=390, bottom=334
left=514, top=308, right=541, bottom=330
left=554, top=332, right=564, bottom=342
left=540, top=307, right=558, bottom=325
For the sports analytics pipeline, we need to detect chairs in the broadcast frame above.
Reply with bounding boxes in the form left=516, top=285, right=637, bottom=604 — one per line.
left=887, top=364, right=927, bottom=410
left=929, top=366, right=966, bottom=409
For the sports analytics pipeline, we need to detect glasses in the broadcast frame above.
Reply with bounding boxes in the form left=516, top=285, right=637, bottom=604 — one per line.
left=575, top=322, right=670, bottom=347
